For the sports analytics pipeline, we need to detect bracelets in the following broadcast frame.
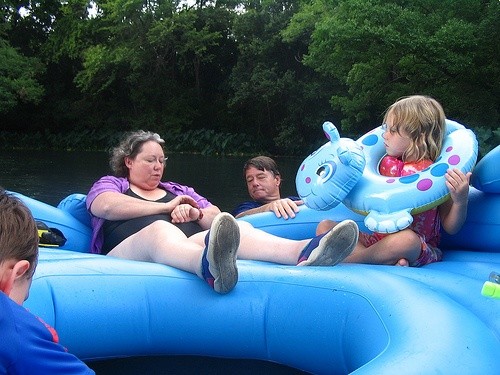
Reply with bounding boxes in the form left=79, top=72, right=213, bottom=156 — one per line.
left=192, top=206, right=203, bottom=224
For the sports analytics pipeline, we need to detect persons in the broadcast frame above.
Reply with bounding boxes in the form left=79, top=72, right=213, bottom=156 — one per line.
left=0, top=188, right=98, bottom=375
left=85, top=131, right=359, bottom=294
left=316, top=96, right=472, bottom=267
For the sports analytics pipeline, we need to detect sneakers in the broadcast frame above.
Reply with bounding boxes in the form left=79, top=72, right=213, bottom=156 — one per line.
left=201, top=211, right=241, bottom=295
left=296, top=218, right=359, bottom=266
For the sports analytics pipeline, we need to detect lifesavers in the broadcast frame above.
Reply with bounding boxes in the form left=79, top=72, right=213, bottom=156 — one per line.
left=291, top=117, right=479, bottom=236
